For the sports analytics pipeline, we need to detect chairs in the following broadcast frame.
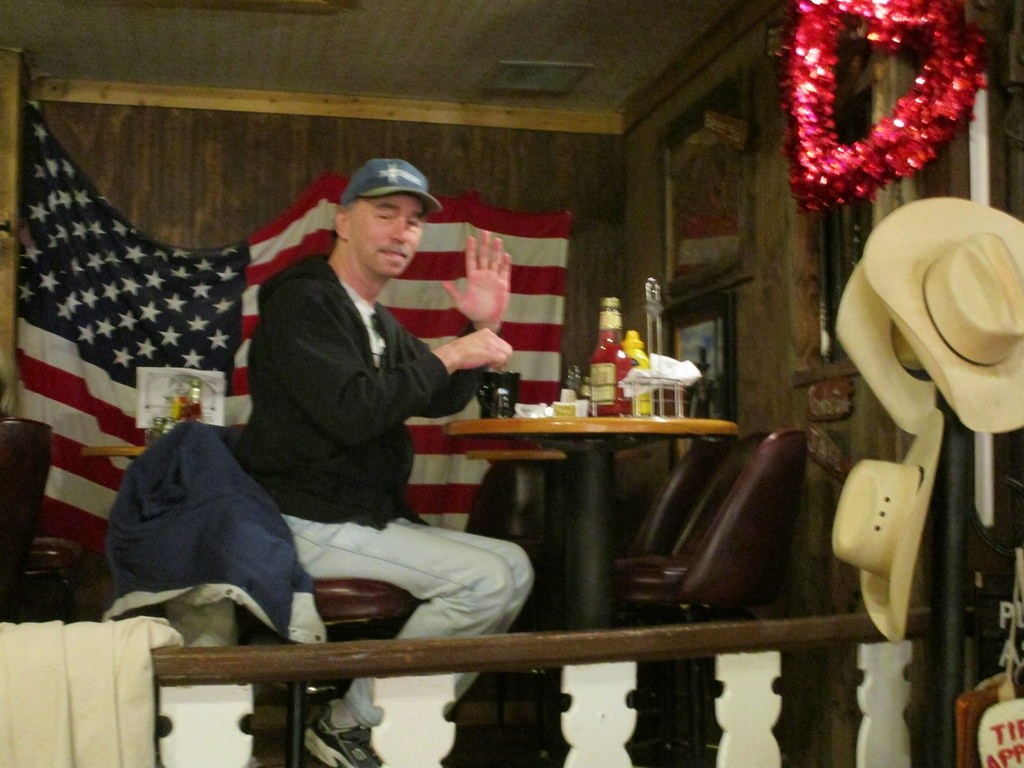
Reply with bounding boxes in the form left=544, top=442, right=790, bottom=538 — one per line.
left=0, top=416, right=412, bottom=768
left=610, top=427, right=809, bottom=768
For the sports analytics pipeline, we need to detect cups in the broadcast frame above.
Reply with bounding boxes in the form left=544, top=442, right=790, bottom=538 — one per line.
left=476, top=372, right=520, bottom=419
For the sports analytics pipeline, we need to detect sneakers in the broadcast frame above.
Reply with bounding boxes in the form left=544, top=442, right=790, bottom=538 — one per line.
left=302, top=704, right=388, bottom=768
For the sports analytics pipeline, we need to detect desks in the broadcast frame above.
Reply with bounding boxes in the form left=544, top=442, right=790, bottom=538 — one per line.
left=449, top=418, right=740, bottom=634
left=465, top=448, right=640, bottom=633
left=80, top=444, right=148, bottom=460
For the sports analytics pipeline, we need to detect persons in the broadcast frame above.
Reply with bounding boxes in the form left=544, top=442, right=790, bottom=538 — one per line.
left=688, top=346, right=720, bottom=419
left=237, top=157, right=535, bottom=768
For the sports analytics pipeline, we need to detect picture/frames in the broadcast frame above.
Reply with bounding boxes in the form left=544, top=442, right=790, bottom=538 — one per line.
left=657, top=64, right=758, bottom=291
left=663, top=289, right=738, bottom=423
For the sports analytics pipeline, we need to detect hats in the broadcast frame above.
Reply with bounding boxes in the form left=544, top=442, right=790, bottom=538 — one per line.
left=835, top=255, right=943, bottom=434
left=831, top=409, right=944, bottom=644
left=862, top=198, right=1024, bottom=434
left=340, top=158, right=442, bottom=212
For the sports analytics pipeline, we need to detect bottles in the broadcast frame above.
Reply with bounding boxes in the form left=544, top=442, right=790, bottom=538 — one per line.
left=589, top=297, right=632, bottom=416
left=621, top=331, right=653, bottom=416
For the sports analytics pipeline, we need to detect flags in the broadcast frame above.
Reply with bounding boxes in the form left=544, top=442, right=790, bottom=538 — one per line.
left=16, top=98, right=570, bottom=558
